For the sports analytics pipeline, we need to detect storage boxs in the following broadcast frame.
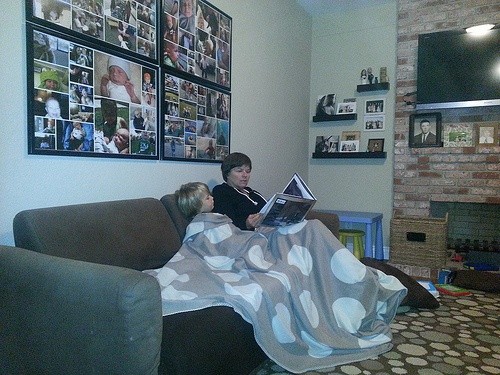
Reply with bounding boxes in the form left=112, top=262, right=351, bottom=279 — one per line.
left=417, top=280, right=439, bottom=296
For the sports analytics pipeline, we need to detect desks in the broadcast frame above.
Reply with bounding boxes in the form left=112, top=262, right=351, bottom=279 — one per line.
left=311, top=209, right=384, bottom=260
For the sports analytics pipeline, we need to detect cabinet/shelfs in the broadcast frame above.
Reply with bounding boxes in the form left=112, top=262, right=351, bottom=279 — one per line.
left=312, top=83, right=389, bottom=159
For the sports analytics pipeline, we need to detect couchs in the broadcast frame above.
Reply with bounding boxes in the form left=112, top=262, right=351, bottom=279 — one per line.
left=0, top=194, right=339, bottom=375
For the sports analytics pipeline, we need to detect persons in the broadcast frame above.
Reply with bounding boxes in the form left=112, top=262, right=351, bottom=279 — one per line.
left=175, top=182, right=214, bottom=222
left=414, top=120, right=436, bottom=144
left=211, top=152, right=269, bottom=231
left=360, top=67, right=374, bottom=84
left=33, top=0, right=231, bottom=159
left=368, top=102, right=382, bottom=112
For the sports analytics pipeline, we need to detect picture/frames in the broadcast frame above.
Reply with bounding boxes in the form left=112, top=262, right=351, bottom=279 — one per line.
left=368, top=139, right=384, bottom=152
left=23, top=0, right=232, bottom=164
left=409, top=112, right=442, bottom=148
left=339, top=140, right=359, bottom=152
left=336, top=102, right=356, bottom=115
left=363, top=98, right=385, bottom=114
left=362, top=115, right=385, bottom=131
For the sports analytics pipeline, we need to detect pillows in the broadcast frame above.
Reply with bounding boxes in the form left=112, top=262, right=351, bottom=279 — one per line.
left=360, top=258, right=440, bottom=309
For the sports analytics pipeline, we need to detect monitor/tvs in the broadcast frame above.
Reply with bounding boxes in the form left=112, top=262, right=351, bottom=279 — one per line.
left=415, top=23, right=500, bottom=111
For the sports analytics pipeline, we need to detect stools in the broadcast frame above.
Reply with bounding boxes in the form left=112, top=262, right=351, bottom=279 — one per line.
left=339, top=229, right=365, bottom=260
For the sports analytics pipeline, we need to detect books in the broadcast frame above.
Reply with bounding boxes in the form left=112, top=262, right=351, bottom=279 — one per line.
left=259, top=172, right=317, bottom=226
